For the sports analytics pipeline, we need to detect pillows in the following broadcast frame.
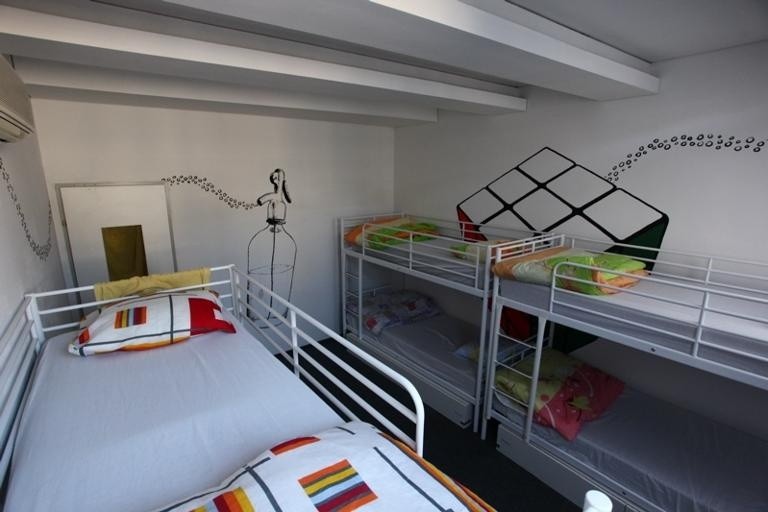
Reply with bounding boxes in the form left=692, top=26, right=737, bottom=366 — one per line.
left=453, top=323, right=524, bottom=365
left=449, top=238, right=548, bottom=263
left=67, top=289, right=237, bottom=355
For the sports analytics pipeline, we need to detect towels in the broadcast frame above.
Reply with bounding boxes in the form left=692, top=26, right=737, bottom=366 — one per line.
left=93, top=266, right=210, bottom=306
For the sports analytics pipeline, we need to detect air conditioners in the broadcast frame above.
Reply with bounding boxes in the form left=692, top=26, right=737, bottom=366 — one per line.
left=0, top=56, right=33, bottom=144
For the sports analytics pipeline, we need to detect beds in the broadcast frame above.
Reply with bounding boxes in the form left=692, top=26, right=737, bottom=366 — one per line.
left=1, top=263, right=614, bottom=512
left=481, top=233, right=768, bottom=511
left=339, top=213, right=556, bottom=434
